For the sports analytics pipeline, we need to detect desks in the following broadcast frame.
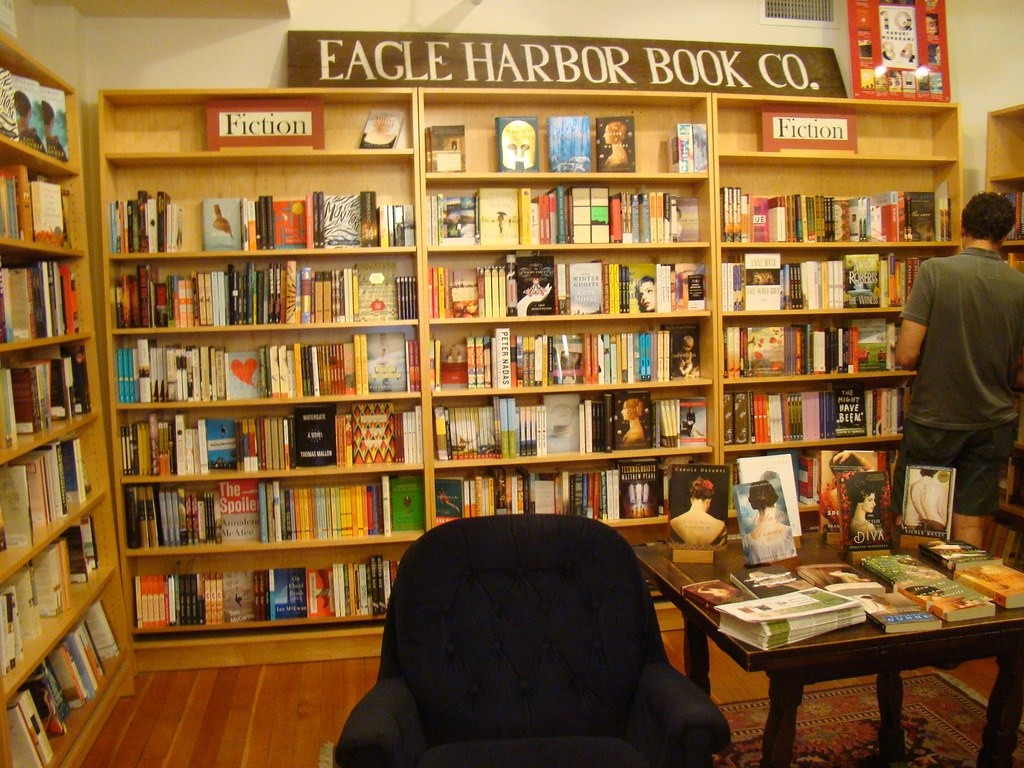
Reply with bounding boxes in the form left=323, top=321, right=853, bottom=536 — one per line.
left=632, top=532, right=1024, bottom=768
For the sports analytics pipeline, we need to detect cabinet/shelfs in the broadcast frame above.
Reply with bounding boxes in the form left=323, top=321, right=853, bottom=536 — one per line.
left=981, top=104, right=1024, bottom=572
left=0, top=34, right=138, bottom=768
left=711, top=92, right=964, bottom=541
left=418, top=88, right=720, bottom=614
left=98, top=88, right=431, bottom=672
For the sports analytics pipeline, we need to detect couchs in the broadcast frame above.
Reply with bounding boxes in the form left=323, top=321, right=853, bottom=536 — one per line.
left=332, top=514, right=730, bottom=768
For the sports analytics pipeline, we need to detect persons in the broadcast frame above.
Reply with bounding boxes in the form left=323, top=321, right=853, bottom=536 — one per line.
left=850, top=481, right=880, bottom=538
left=746, top=480, right=797, bottom=565
left=904, top=586, right=973, bottom=611
left=669, top=477, right=728, bottom=551
left=635, top=276, right=657, bottom=312
left=687, top=406, right=696, bottom=437
left=749, top=567, right=814, bottom=591
left=890, top=192, right=1024, bottom=548
left=926, top=15, right=938, bottom=34
left=697, top=584, right=734, bottom=600
left=819, top=451, right=874, bottom=533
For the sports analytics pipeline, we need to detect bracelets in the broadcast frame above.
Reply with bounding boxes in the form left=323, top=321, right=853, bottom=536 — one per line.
left=859, top=457, right=865, bottom=465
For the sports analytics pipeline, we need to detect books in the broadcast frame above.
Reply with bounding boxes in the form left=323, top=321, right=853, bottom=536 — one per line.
left=0, top=67, right=120, bottom=768
left=108, top=191, right=424, bottom=629
left=119, top=403, right=424, bottom=476
left=666, top=450, right=1023, bottom=652
left=426, top=185, right=708, bottom=590
left=424, top=116, right=707, bottom=175
left=359, top=109, right=404, bottom=149
left=995, top=192, right=1024, bottom=273
left=720, top=186, right=953, bottom=506
